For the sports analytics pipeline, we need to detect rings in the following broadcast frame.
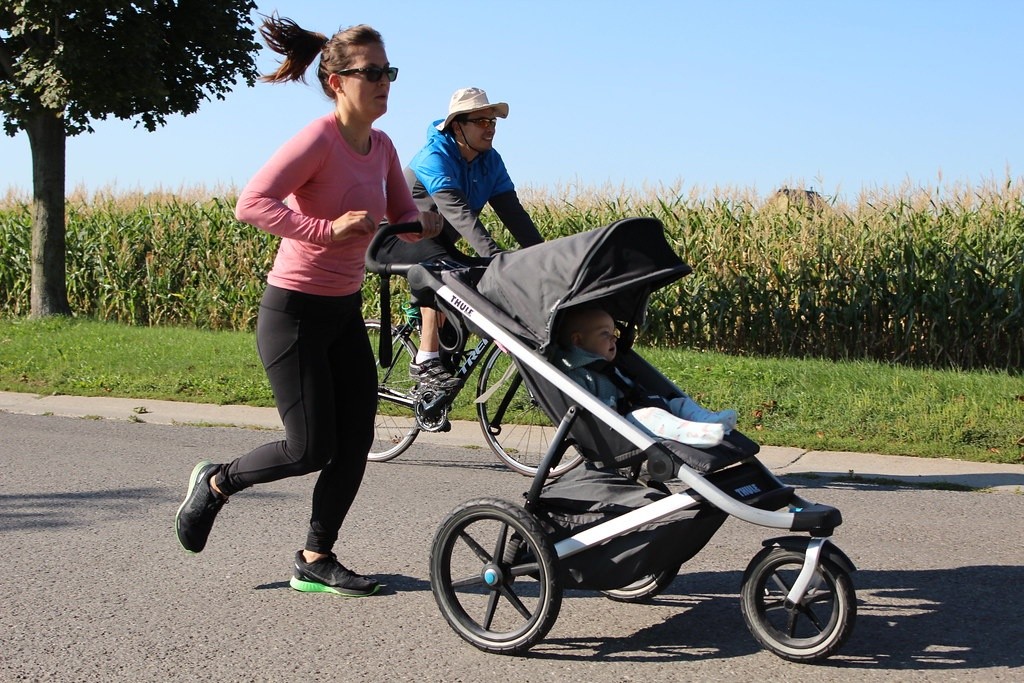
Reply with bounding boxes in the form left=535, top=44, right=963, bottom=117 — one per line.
left=436, top=220, right=441, bottom=227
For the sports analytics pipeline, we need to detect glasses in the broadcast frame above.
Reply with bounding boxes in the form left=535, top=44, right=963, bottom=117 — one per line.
left=334, top=64, right=400, bottom=85
left=466, top=116, right=496, bottom=129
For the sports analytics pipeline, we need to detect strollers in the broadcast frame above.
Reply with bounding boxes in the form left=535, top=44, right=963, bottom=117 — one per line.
left=364, top=216, right=858, bottom=660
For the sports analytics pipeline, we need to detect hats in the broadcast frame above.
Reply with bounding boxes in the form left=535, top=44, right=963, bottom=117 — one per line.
left=434, top=88, right=509, bottom=140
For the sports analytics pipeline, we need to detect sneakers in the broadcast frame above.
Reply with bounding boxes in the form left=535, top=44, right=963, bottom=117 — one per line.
left=288, top=550, right=382, bottom=596
left=408, top=357, right=462, bottom=391
left=177, top=462, right=229, bottom=553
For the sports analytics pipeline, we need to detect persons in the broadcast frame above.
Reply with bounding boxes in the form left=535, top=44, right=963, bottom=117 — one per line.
left=397, top=87, right=547, bottom=430
left=569, top=307, right=739, bottom=446
left=176, top=7, right=442, bottom=597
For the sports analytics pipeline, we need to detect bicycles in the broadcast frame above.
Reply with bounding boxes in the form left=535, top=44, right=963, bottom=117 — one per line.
left=364, top=306, right=586, bottom=477
